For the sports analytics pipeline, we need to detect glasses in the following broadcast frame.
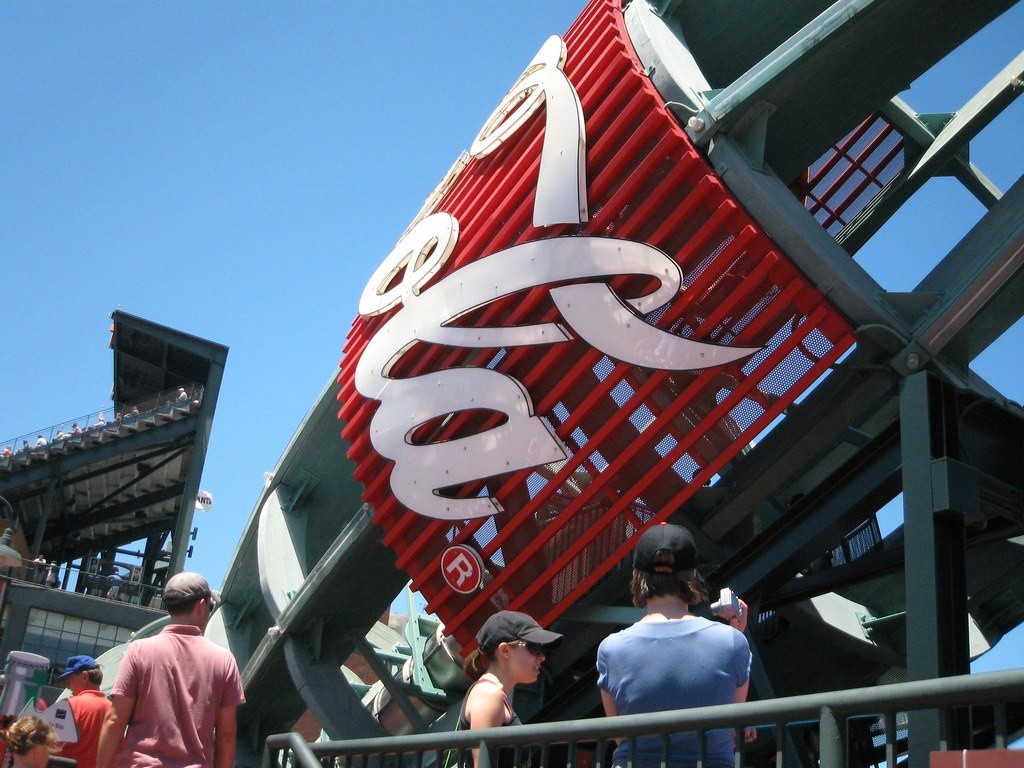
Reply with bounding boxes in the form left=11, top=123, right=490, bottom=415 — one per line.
left=203, top=597, right=215, bottom=612
left=506, top=640, right=549, bottom=657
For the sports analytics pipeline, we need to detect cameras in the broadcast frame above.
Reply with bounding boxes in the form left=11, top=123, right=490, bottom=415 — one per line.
left=710, top=587, right=744, bottom=622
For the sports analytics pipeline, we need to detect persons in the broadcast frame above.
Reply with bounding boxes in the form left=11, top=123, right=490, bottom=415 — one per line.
left=97, top=573, right=246, bottom=768
left=194, top=389, right=199, bottom=397
left=107, top=571, right=123, bottom=600
left=597, top=522, right=757, bottom=768
left=0, top=716, right=57, bottom=768
left=458, top=611, right=564, bottom=768
left=176, top=388, right=187, bottom=403
left=0, top=406, right=139, bottom=457
left=33, top=555, right=47, bottom=584
left=49, top=655, right=124, bottom=768
left=45, top=560, right=57, bottom=586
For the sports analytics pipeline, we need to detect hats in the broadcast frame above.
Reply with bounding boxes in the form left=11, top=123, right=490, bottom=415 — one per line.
left=632, top=522, right=698, bottom=574
left=56, top=654, right=98, bottom=682
left=476, top=610, right=566, bottom=652
left=163, top=572, right=221, bottom=607
